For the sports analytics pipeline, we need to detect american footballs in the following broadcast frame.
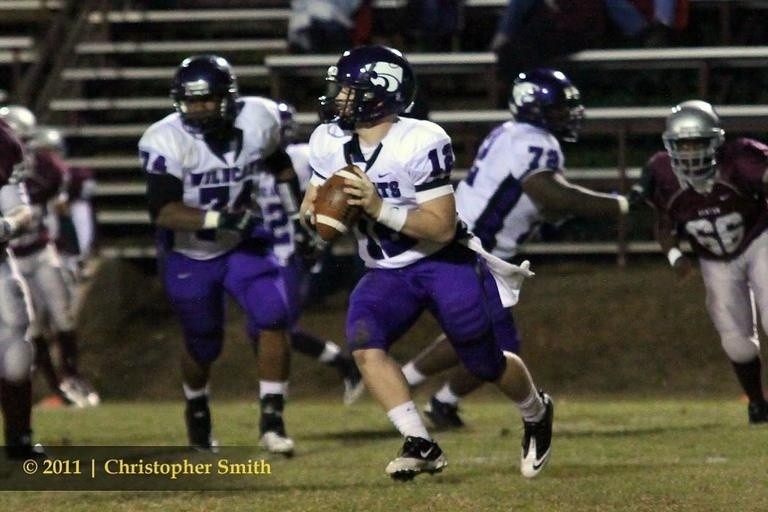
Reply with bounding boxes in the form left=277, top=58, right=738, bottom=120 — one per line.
left=315, top=165, right=364, bottom=242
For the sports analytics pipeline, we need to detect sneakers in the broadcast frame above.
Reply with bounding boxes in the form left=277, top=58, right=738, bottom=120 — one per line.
left=748, top=400, right=767, bottom=425
left=71, top=377, right=98, bottom=406
left=425, top=398, right=464, bottom=429
left=386, top=437, right=445, bottom=479
left=184, top=407, right=216, bottom=454
left=259, top=418, right=292, bottom=454
left=519, top=393, right=553, bottom=477
left=11, top=443, right=51, bottom=464
left=343, top=369, right=366, bottom=405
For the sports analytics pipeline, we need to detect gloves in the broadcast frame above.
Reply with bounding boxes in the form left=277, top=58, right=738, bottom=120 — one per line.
left=215, top=212, right=258, bottom=235
left=623, top=187, right=651, bottom=213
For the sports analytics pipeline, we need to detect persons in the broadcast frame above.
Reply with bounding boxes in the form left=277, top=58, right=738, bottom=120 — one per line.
left=138, top=56, right=292, bottom=457
left=8, top=105, right=93, bottom=388
left=31, top=127, right=99, bottom=353
left=404, top=70, right=628, bottom=426
left=248, top=101, right=364, bottom=405
left=286, top=0, right=698, bottom=48
left=300, top=47, right=553, bottom=478
left=0, top=123, right=46, bottom=469
left=633, top=100, right=768, bottom=422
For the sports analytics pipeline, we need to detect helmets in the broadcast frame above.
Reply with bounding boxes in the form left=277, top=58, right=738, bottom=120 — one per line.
left=170, top=53, right=239, bottom=124
left=319, top=46, right=414, bottom=120
left=508, top=71, right=584, bottom=143
left=663, top=102, right=725, bottom=180
left=1, top=106, right=38, bottom=143
left=34, top=128, right=68, bottom=155
